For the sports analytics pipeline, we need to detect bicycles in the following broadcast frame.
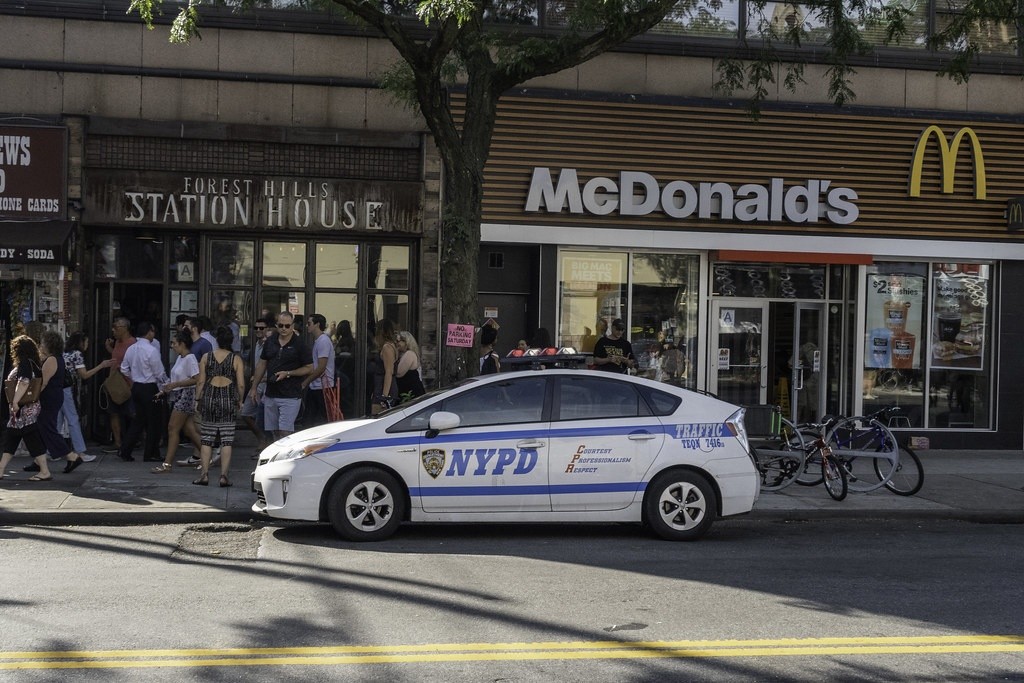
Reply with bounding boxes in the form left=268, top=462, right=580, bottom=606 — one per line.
left=878, top=369, right=903, bottom=391
left=755, top=418, right=847, bottom=502
left=779, top=406, right=925, bottom=496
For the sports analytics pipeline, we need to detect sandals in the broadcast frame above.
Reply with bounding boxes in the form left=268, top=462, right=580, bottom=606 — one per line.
left=192, top=471, right=208, bottom=486
left=220, top=475, right=233, bottom=487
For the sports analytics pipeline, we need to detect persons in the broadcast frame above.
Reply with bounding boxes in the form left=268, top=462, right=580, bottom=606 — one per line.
left=0, top=312, right=427, bottom=487
left=787, top=327, right=818, bottom=424
left=479, top=324, right=551, bottom=404
left=594, top=318, right=639, bottom=398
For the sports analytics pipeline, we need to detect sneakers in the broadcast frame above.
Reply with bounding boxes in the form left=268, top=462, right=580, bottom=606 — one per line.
left=100, top=445, right=120, bottom=454
left=210, top=448, right=221, bottom=465
left=177, top=456, right=201, bottom=465
left=80, top=452, right=96, bottom=462
left=46, top=454, right=61, bottom=462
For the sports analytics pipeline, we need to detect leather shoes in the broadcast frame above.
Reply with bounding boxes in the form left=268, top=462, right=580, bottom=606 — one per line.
left=23, top=462, right=50, bottom=472
left=62, top=457, right=84, bottom=473
left=121, top=448, right=136, bottom=462
left=143, top=455, right=166, bottom=462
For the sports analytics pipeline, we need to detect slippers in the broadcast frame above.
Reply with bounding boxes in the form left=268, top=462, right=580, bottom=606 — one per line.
left=28, top=475, right=54, bottom=481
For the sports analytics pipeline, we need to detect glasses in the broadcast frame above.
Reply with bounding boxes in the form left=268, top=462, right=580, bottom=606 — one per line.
left=394, top=338, right=405, bottom=344
left=278, top=322, right=294, bottom=329
left=112, top=325, right=125, bottom=331
left=254, top=326, right=268, bottom=330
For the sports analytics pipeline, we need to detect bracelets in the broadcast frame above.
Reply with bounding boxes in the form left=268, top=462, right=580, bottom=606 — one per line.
left=160, top=390, right=164, bottom=395
left=286, top=371, right=289, bottom=378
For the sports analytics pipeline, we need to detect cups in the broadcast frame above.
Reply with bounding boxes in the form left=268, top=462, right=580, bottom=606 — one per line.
left=868, top=327, right=893, bottom=368
left=936, top=313, right=962, bottom=343
left=890, top=332, right=916, bottom=369
left=884, top=300, right=908, bottom=336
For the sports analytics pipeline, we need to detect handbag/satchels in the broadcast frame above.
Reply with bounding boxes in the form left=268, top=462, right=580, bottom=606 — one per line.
left=98, top=367, right=133, bottom=409
left=4, top=358, right=43, bottom=404
left=63, top=369, right=73, bottom=389
left=480, top=349, right=498, bottom=375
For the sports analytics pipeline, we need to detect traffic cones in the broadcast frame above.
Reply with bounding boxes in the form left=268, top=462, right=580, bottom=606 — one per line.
left=776, top=377, right=792, bottom=422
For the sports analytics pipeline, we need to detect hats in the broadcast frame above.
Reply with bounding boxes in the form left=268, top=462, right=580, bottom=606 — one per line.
left=612, top=318, right=626, bottom=330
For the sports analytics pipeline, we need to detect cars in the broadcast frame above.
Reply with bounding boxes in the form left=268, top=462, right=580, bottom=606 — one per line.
left=629, top=340, right=657, bottom=370
left=251, top=369, right=761, bottom=543
left=734, top=357, right=762, bottom=387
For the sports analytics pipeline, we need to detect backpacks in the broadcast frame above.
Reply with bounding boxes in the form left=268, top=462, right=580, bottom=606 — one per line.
left=798, top=347, right=814, bottom=381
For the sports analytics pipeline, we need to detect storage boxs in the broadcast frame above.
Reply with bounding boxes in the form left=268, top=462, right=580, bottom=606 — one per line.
left=746, top=405, right=782, bottom=438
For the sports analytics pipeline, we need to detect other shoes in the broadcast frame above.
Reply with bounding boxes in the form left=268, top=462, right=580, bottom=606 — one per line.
left=193, top=459, right=215, bottom=472
left=151, top=462, right=173, bottom=474
left=251, top=449, right=264, bottom=460
